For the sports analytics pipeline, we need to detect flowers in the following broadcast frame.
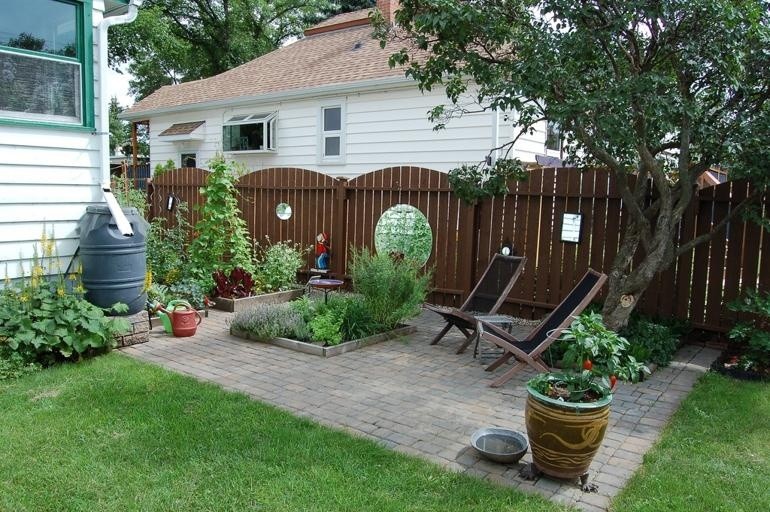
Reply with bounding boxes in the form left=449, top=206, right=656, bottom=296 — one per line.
left=527, top=309, right=652, bottom=400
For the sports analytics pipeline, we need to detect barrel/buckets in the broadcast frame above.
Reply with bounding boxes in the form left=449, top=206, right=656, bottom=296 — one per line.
left=80, top=205, right=148, bottom=316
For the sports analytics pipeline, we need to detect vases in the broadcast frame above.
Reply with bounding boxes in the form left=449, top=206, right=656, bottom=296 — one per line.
left=518, top=376, right=612, bottom=492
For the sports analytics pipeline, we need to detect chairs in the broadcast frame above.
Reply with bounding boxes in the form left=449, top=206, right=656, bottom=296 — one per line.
left=424, top=253, right=529, bottom=354
left=477, top=268, right=608, bottom=387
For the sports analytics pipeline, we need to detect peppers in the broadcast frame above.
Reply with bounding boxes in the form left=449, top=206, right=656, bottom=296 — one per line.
left=610, top=376, right=616, bottom=390
left=585, top=360, right=591, bottom=369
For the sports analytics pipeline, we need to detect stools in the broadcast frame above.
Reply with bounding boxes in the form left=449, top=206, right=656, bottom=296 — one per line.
left=473, top=316, right=513, bottom=359
